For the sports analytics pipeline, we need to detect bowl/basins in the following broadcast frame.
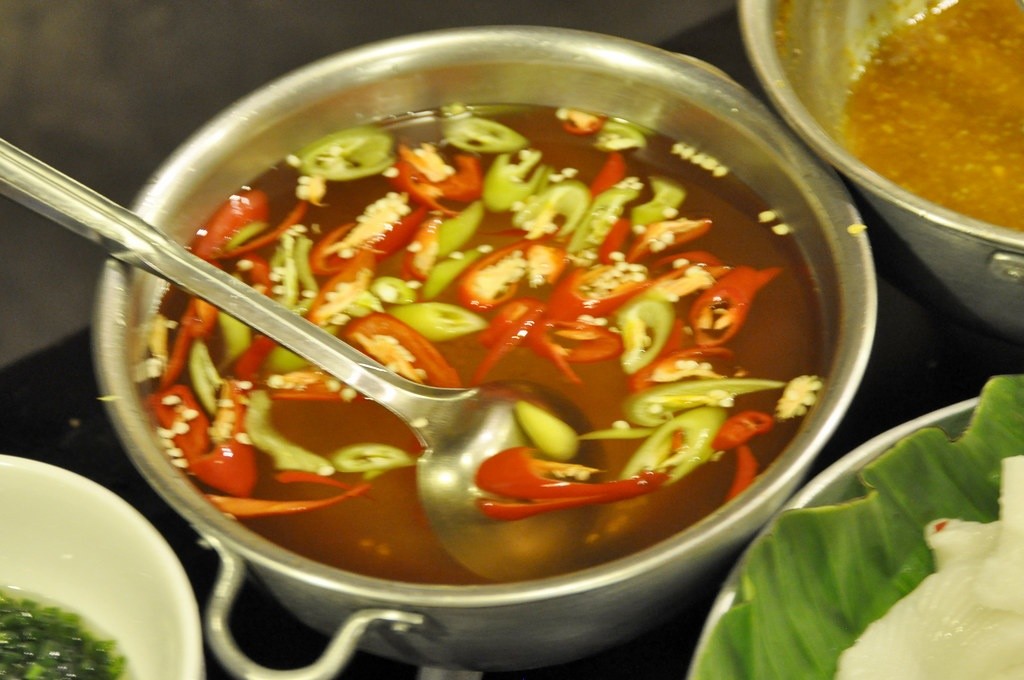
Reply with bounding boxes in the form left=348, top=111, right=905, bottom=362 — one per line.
left=0, top=454, right=210, bottom=680
left=686, top=398, right=977, bottom=680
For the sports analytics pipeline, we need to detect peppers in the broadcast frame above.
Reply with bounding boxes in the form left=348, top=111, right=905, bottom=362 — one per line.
left=127, top=112, right=821, bottom=519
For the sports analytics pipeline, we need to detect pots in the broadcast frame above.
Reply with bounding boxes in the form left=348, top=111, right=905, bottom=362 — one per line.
left=93, top=24, right=878, bottom=680
left=736, top=0, right=1024, bottom=348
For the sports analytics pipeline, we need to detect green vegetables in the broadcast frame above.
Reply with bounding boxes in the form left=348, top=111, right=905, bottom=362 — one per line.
left=0, top=590, right=129, bottom=680
